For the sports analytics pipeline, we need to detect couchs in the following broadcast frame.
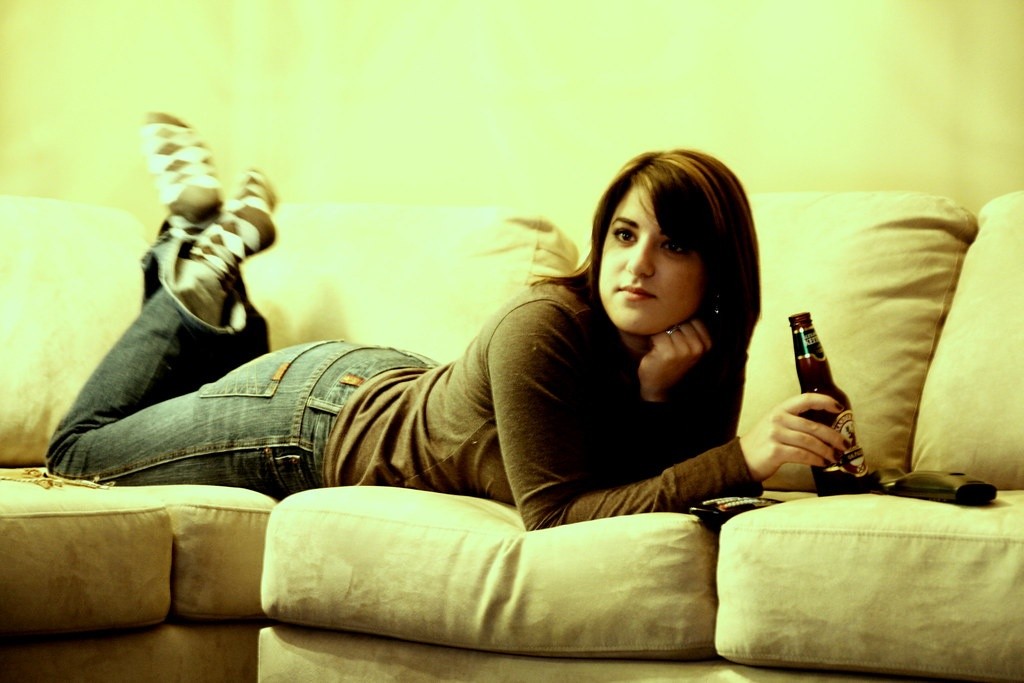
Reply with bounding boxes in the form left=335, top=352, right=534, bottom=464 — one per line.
left=0, top=188, right=1024, bottom=683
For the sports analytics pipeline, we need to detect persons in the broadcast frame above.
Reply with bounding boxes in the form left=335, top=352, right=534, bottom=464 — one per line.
left=46, top=112, right=853, bottom=533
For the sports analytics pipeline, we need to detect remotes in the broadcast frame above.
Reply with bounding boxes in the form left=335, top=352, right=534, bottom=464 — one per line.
left=879, top=470, right=998, bottom=504
left=691, top=496, right=785, bottom=519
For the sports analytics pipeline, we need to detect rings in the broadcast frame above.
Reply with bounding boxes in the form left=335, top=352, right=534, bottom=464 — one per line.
left=666, top=327, right=680, bottom=334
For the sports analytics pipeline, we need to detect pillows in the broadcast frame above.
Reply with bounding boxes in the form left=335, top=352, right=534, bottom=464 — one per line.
left=0, top=193, right=146, bottom=465
left=910, top=191, right=1024, bottom=489
left=720, top=190, right=978, bottom=488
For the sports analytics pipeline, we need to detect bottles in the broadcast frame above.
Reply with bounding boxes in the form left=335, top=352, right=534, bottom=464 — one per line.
left=789, top=312, right=871, bottom=497
